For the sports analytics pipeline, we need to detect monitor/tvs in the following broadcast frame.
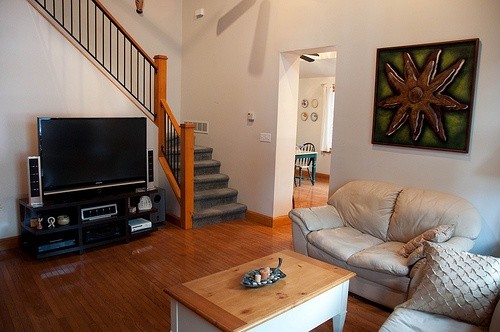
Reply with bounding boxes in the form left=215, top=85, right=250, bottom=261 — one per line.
left=37, top=115, right=148, bottom=195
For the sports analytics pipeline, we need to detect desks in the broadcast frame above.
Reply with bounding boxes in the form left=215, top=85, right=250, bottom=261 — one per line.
left=295, top=151, right=317, bottom=187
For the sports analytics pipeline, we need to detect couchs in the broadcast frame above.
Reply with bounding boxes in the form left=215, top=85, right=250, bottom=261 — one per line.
left=378, top=263, right=500, bottom=332
left=288, top=179, right=480, bottom=309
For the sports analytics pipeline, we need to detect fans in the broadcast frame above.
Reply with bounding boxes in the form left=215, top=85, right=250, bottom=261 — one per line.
left=300, top=52, right=320, bottom=63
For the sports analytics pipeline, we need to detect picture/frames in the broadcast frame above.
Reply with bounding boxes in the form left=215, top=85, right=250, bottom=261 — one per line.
left=371, top=38, right=480, bottom=154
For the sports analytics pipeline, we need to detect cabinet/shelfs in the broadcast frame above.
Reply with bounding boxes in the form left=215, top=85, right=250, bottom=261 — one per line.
left=15, top=193, right=155, bottom=262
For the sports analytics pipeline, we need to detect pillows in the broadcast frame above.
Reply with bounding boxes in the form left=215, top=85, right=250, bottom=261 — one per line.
left=404, top=242, right=500, bottom=328
left=400, top=222, right=455, bottom=257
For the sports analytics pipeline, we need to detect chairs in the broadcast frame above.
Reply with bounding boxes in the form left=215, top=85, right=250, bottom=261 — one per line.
left=299, top=143, right=315, bottom=185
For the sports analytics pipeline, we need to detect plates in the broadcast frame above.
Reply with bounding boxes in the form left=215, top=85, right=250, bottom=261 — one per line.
left=240, top=267, right=286, bottom=288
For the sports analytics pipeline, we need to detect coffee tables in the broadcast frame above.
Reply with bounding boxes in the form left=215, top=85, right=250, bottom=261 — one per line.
left=163, top=249, right=357, bottom=332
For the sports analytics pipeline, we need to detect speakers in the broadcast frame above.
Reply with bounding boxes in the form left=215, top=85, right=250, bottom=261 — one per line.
left=27, top=156, right=42, bottom=207
left=147, top=149, right=156, bottom=190
left=149, top=187, right=165, bottom=226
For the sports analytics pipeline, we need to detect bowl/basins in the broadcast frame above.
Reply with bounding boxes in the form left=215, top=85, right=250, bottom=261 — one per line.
left=57, top=216, right=70, bottom=225
